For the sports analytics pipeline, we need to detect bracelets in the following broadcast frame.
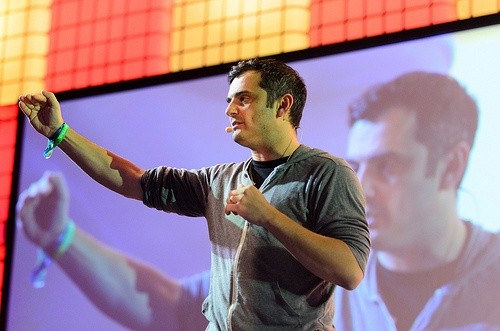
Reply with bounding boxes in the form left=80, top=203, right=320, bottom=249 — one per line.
left=29, top=220, right=75, bottom=288
left=42, top=123, right=72, bottom=160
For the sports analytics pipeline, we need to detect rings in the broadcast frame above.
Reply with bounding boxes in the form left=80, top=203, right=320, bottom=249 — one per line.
left=230, top=196, right=233, bottom=204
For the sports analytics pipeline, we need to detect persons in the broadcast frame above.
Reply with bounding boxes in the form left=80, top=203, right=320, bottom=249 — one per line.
left=18, top=57, right=371, bottom=331
left=16, top=70, right=500, bottom=331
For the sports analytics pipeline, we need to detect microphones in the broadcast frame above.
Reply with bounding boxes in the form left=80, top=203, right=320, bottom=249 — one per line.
left=225, top=127, right=233, bottom=133
